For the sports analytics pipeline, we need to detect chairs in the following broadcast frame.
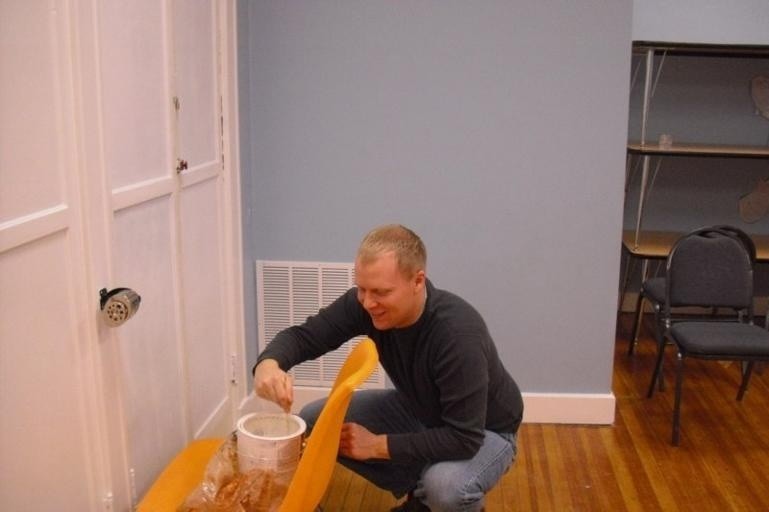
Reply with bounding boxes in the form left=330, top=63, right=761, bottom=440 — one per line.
left=134, top=336, right=379, bottom=512
left=629, top=223, right=769, bottom=447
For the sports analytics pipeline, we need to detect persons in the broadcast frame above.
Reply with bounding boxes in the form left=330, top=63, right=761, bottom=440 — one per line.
left=252, top=223, right=525, bottom=510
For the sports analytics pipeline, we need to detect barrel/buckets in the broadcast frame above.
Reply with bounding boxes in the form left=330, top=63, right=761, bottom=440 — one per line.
left=233, top=383, right=308, bottom=486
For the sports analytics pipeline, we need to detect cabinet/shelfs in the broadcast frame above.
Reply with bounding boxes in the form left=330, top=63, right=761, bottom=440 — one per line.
left=614, top=38, right=769, bottom=357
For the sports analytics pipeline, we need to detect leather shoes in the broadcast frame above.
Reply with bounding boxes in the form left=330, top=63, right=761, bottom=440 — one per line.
left=389, top=494, right=429, bottom=512
